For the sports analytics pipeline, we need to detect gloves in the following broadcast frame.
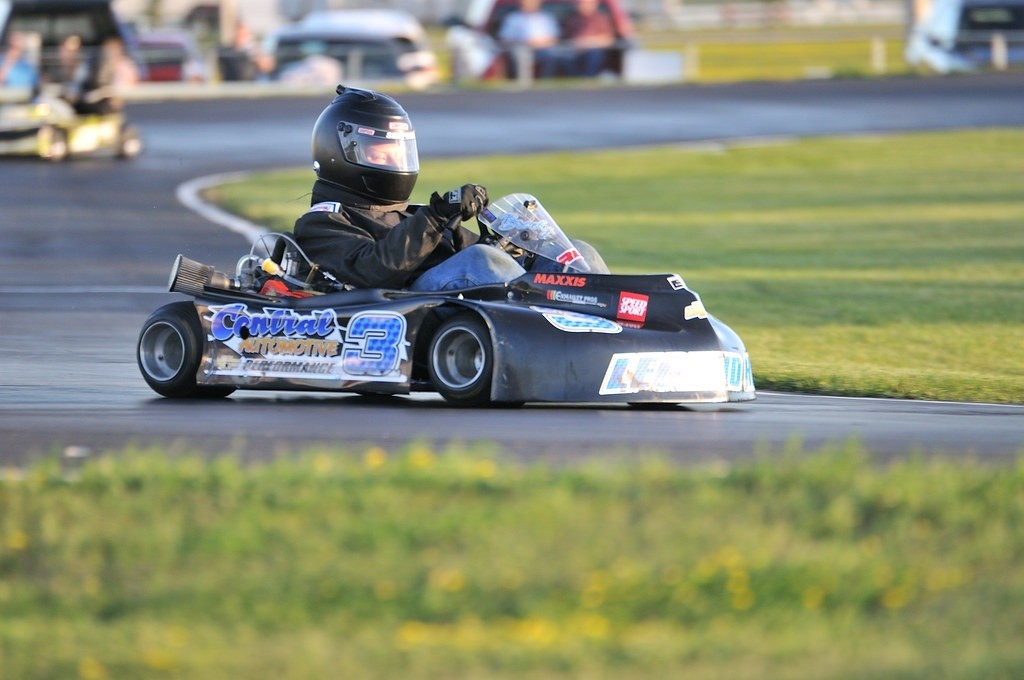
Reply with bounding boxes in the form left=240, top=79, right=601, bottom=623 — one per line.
left=434, top=184, right=489, bottom=222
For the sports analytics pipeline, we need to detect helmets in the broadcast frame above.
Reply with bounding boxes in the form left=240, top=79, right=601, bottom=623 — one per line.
left=312, top=84, right=420, bottom=203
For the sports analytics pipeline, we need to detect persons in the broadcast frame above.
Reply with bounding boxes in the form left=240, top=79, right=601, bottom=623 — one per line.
left=293, top=85, right=613, bottom=290
left=0, top=27, right=139, bottom=116
left=497, top=1, right=619, bottom=81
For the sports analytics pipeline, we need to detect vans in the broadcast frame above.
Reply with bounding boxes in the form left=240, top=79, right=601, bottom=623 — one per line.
left=265, top=12, right=434, bottom=85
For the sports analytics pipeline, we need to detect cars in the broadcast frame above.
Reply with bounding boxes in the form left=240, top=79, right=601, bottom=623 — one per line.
left=130, top=27, right=221, bottom=83
left=450, top=0, right=635, bottom=88
left=906, top=0, right=1024, bottom=70
